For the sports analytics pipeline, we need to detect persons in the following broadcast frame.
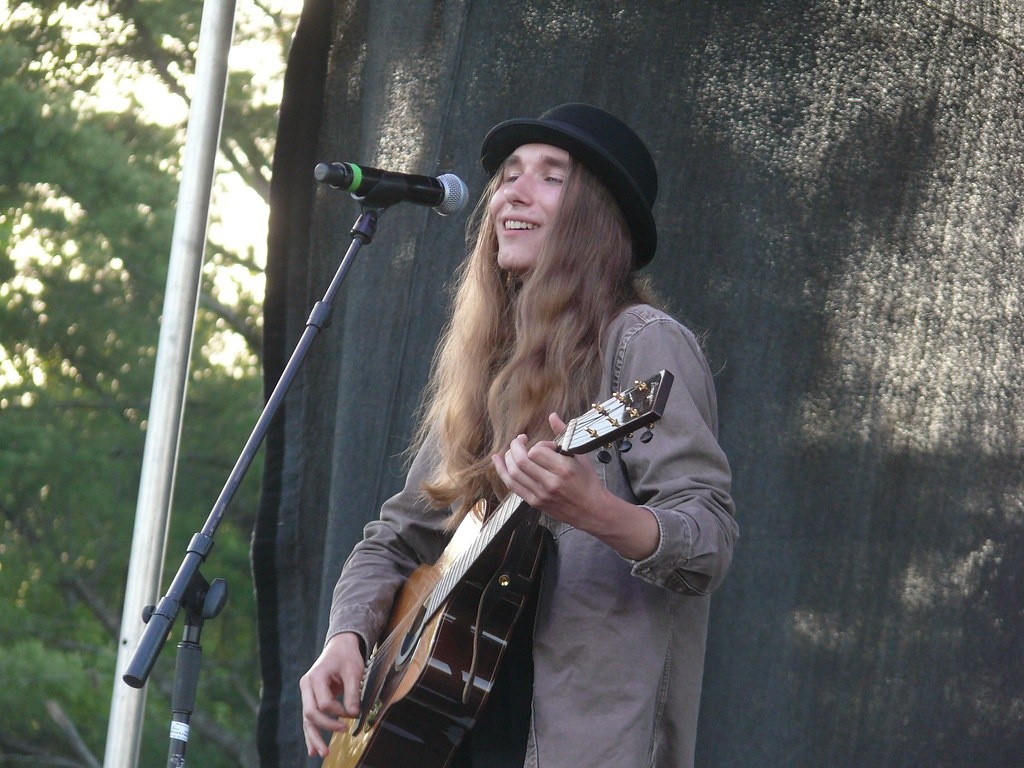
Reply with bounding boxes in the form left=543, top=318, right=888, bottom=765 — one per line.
left=300, top=103, right=739, bottom=767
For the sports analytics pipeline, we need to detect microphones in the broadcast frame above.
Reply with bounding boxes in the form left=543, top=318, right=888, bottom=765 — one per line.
left=314, top=162, right=469, bottom=217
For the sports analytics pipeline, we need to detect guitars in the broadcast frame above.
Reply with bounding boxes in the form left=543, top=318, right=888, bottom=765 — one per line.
left=321, top=366, right=673, bottom=768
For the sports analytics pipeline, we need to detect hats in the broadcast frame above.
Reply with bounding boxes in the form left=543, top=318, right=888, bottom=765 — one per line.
left=481, top=103, right=657, bottom=271
